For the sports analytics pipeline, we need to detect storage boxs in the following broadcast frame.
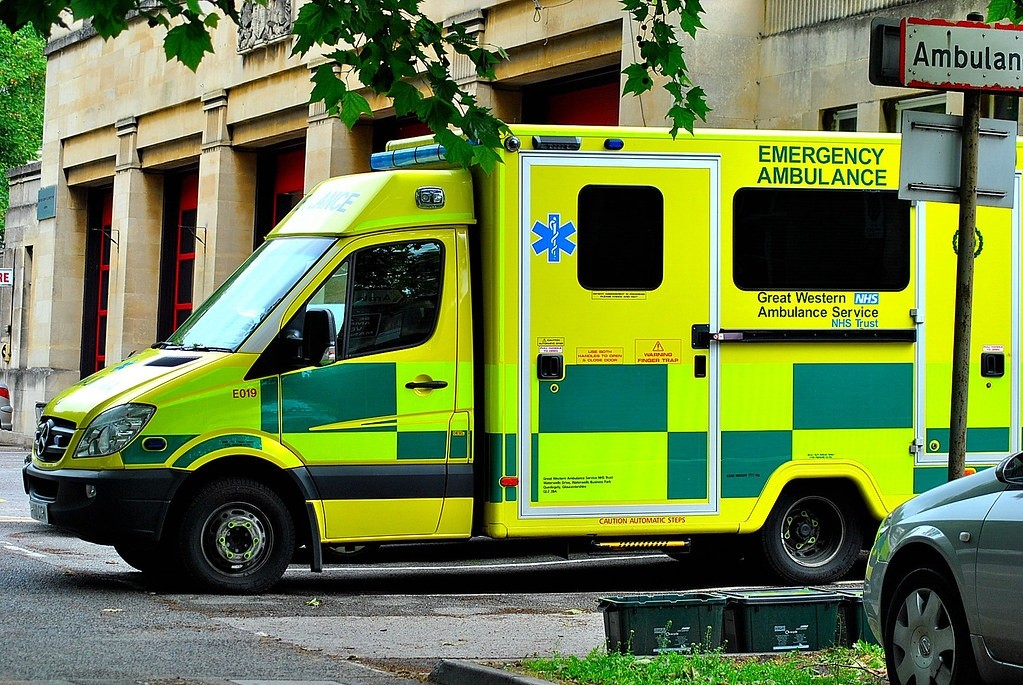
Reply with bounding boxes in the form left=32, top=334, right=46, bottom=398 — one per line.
left=595, top=592, right=729, bottom=656
left=837, top=588, right=882, bottom=648
left=716, top=589, right=845, bottom=652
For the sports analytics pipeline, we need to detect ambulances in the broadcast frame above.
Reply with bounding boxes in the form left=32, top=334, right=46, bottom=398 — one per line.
left=20, top=122, right=1023, bottom=598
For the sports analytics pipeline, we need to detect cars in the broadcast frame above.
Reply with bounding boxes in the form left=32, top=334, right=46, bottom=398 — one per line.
left=862, top=449, right=1023, bottom=685
